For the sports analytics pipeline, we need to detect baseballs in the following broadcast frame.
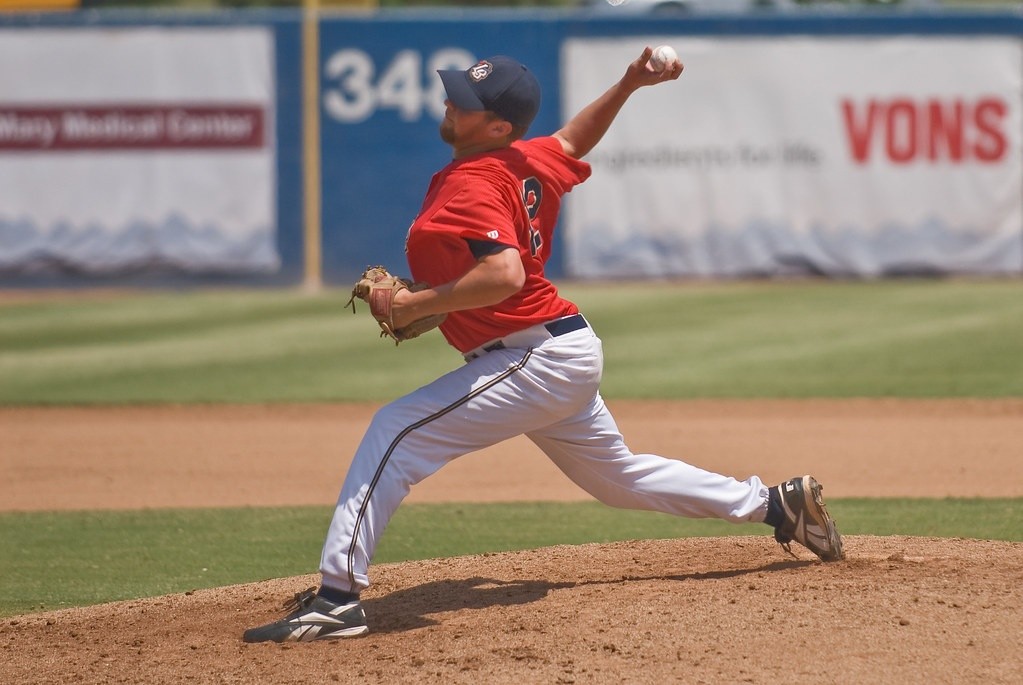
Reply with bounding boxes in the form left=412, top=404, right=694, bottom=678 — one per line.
left=650, top=44, right=678, bottom=74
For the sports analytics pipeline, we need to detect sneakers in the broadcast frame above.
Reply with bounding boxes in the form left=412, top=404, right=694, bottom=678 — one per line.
left=241, top=586, right=370, bottom=643
left=779, top=473, right=843, bottom=560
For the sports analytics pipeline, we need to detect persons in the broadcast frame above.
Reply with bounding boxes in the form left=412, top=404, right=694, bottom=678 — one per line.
left=242, top=46, right=844, bottom=643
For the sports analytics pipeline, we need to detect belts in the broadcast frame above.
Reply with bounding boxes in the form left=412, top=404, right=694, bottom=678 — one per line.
left=467, top=313, right=588, bottom=360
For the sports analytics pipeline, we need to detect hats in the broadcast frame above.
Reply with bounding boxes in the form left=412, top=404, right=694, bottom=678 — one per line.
left=436, top=55, right=540, bottom=130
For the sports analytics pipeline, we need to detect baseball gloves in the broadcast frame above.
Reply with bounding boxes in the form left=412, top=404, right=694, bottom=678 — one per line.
left=350, top=264, right=451, bottom=347
